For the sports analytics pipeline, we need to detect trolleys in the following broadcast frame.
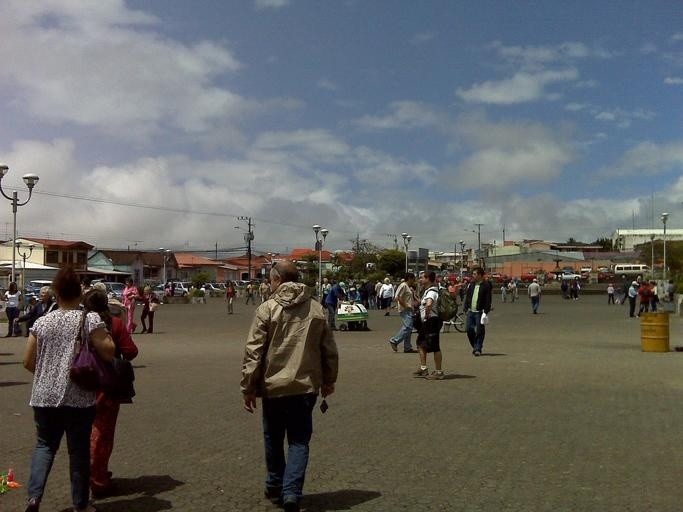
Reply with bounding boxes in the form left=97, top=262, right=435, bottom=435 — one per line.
left=336, top=301, right=368, bottom=332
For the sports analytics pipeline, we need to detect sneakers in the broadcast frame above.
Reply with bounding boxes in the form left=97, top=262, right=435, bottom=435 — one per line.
left=388, top=340, right=397, bottom=352
left=413, top=368, right=445, bottom=379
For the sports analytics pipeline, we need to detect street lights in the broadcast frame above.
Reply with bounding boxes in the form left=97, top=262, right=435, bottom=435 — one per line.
left=312, top=225, right=328, bottom=304
left=650, top=234, right=655, bottom=281
left=658, top=212, right=670, bottom=282
left=15, top=240, right=35, bottom=299
left=0, top=164, right=38, bottom=293
left=402, top=231, right=412, bottom=273
left=458, top=239, right=464, bottom=281
left=266, top=251, right=275, bottom=270
left=158, top=247, right=171, bottom=284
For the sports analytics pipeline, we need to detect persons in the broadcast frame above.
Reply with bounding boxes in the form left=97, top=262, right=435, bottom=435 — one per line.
left=411, top=270, right=445, bottom=378
left=666, top=280, right=674, bottom=302
left=561, top=275, right=583, bottom=301
left=462, top=267, right=492, bottom=357
left=240, top=261, right=339, bottom=511
left=320, top=277, right=394, bottom=330
left=0, top=278, right=272, bottom=338
left=607, top=284, right=616, bottom=304
left=388, top=271, right=466, bottom=353
left=508, top=280, right=516, bottom=302
left=500, top=284, right=511, bottom=303
left=82, top=288, right=139, bottom=499
left=619, top=274, right=659, bottom=318
left=23, top=264, right=115, bottom=512
left=528, top=279, right=541, bottom=314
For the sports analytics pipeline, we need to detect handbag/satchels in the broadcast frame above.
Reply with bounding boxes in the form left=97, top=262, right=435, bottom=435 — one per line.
left=148, top=302, right=160, bottom=312
left=107, top=357, right=136, bottom=404
left=71, top=349, right=104, bottom=388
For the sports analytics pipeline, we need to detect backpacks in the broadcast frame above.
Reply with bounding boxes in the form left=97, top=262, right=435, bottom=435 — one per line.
left=425, top=288, right=458, bottom=320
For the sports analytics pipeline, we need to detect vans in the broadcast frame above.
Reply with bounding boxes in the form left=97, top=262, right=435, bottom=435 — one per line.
left=26, top=278, right=52, bottom=294
left=613, top=263, right=650, bottom=280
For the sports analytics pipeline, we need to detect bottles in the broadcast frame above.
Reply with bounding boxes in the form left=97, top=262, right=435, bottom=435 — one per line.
left=0, top=465, right=14, bottom=494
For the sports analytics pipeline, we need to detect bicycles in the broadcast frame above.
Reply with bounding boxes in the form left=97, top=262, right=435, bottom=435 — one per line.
left=410, top=305, right=466, bottom=334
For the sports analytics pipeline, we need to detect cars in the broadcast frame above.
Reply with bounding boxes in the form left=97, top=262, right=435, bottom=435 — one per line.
left=88, top=276, right=262, bottom=305
left=406, top=265, right=616, bottom=287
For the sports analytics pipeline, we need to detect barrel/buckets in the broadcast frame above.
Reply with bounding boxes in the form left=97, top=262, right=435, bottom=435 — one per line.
left=639, top=310, right=670, bottom=354
